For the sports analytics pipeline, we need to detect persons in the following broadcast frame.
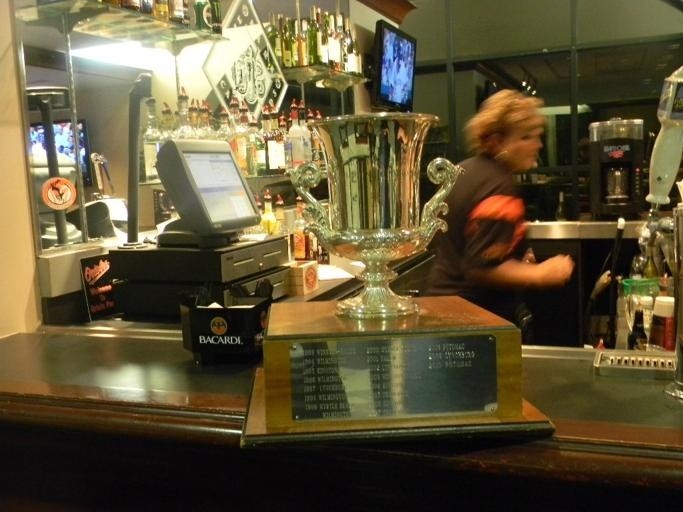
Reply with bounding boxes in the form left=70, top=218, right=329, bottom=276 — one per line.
left=425, top=88, right=575, bottom=345
left=384, top=31, right=414, bottom=103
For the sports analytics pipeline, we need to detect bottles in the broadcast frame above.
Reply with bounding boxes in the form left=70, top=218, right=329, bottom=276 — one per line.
left=554, top=191, right=569, bottom=222
left=260, top=0, right=361, bottom=76
left=107, top=0, right=222, bottom=40
left=625, top=295, right=653, bottom=351
left=152, top=83, right=327, bottom=182
left=642, top=247, right=658, bottom=280
left=249, top=186, right=331, bottom=267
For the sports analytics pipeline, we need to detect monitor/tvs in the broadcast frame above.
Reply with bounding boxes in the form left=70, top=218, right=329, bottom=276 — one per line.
left=155, top=139, right=263, bottom=246
left=25, top=119, right=92, bottom=187
left=360, top=17, right=419, bottom=114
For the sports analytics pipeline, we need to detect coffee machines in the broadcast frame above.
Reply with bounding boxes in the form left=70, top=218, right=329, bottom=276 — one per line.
left=586, top=116, right=647, bottom=220
